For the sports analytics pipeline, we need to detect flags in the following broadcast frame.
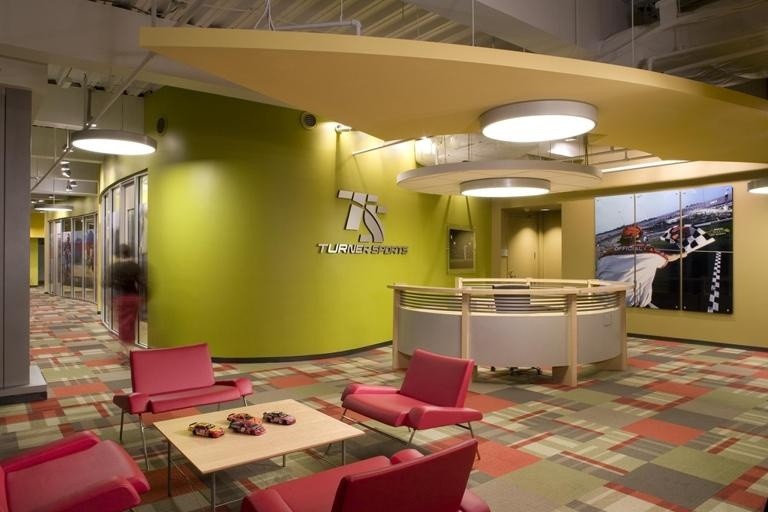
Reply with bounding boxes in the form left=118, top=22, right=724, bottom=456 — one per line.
left=660, top=224, right=715, bottom=253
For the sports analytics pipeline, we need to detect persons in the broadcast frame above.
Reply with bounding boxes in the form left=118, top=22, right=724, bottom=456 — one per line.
left=101, top=242, right=149, bottom=368
left=596, top=223, right=688, bottom=308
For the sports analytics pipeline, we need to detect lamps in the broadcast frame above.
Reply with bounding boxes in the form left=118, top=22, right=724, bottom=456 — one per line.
left=68, top=94, right=157, bottom=157
left=34, top=128, right=74, bottom=212
left=747, top=178, right=768, bottom=194
left=478, top=99, right=599, bottom=146
left=459, top=177, right=552, bottom=198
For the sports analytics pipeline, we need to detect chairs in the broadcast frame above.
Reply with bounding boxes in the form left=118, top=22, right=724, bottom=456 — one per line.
left=491, top=284, right=545, bottom=377
